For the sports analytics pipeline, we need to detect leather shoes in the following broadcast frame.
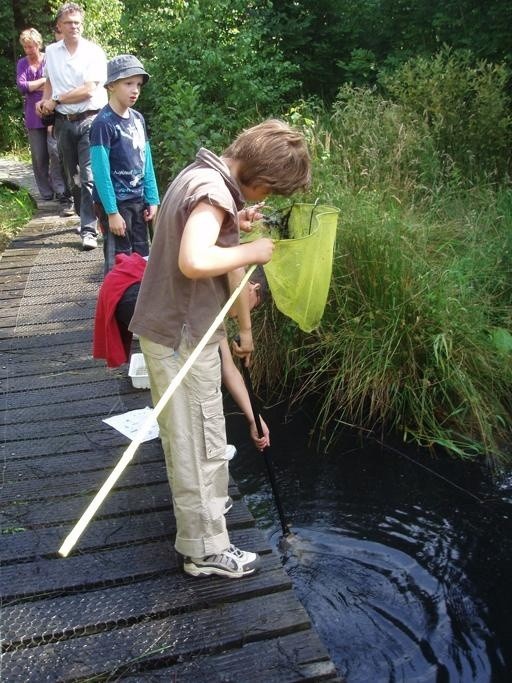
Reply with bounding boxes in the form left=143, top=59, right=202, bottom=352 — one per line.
left=40, top=192, right=67, bottom=201
left=55, top=110, right=98, bottom=122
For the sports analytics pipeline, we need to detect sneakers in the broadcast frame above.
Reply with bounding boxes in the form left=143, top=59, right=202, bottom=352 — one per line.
left=221, top=495, right=234, bottom=515
left=81, top=216, right=101, bottom=251
left=216, top=445, right=237, bottom=463
left=58, top=193, right=75, bottom=217
left=183, top=544, right=262, bottom=579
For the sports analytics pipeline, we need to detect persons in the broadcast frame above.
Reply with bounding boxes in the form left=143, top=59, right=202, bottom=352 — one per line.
left=128, top=123, right=309, bottom=578
left=15, top=27, right=67, bottom=200
left=35, top=1, right=110, bottom=249
left=92, top=251, right=270, bottom=369
left=89, top=55, right=160, bottom=278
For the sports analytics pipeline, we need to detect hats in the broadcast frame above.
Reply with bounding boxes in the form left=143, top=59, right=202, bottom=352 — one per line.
left=103, top=54, right=149, bottom=88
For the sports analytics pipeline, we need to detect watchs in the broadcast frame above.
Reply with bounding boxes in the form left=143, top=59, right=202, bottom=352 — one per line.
left=53, top=95, right=61, bottom=104
